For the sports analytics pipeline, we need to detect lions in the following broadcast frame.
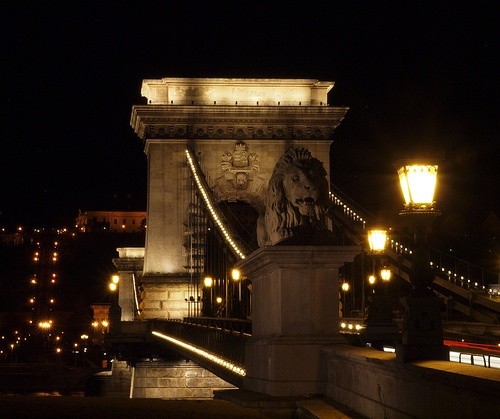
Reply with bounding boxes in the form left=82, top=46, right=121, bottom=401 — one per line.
left=257, top=148, right=331, bottom=246
